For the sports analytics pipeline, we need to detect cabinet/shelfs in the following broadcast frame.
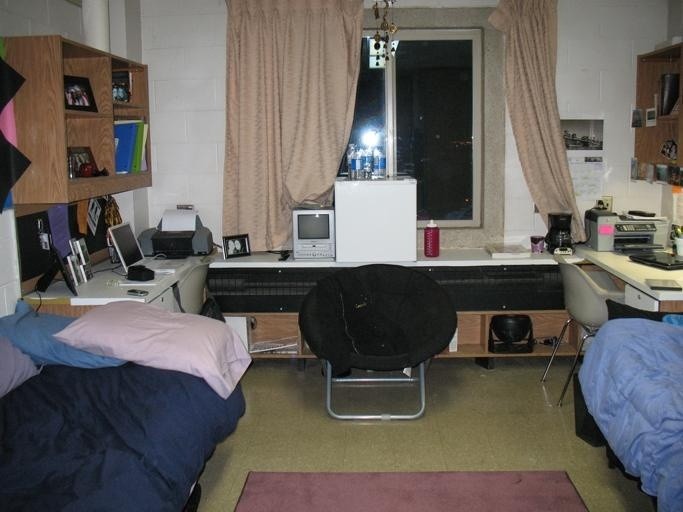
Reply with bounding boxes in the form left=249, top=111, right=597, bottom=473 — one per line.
left=632, top=41, right=683, bottom=188
left=2, top=35, right=152, bottom=203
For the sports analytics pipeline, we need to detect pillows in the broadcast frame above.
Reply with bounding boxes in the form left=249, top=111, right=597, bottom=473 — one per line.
left=0, top=334, right=44, bottom=398
left=0, top=299, right=130, bottom=369
left=51, top=300, right=253, bottom=400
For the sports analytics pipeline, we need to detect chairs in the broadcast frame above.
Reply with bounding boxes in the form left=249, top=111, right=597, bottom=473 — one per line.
left=539, top=253, right=624, bottom=408
left=298, top=262, right=459, bottom=421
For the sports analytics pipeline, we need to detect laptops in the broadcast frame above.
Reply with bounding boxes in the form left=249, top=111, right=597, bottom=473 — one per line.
left=107, top=222, right=183, bottom=273
left=630, top=252, right=683, bottom=270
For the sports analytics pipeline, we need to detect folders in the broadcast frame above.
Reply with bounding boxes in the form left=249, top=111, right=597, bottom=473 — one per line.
left=114, top=119, right=148, bottom=174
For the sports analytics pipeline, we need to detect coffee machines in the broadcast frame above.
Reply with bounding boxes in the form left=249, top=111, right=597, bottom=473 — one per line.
left=545, top=212, right=576, bottom=255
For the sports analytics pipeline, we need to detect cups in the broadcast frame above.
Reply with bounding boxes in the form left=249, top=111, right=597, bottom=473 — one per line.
left=660, top=71, right=679, bottom=114
left=530, top=235, right=544, bottom=254
left=672, top=239, right=683, bottom=256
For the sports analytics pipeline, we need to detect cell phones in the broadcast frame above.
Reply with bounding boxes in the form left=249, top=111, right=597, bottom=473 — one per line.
left=127, top=289, right=147, bottom=296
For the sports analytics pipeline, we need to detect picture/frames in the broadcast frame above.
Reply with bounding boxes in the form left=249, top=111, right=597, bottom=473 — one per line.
left=221, top=235, right=253, bottom=260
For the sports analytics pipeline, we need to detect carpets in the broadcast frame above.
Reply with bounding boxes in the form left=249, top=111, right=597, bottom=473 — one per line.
left=233, top=470, right=590, bottom=512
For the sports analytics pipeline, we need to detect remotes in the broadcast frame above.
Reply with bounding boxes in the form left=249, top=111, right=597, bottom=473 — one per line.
left=628, top=210, right=655, bottom=217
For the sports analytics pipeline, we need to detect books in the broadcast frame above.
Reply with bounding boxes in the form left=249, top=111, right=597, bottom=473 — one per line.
left=484, top=244, right=529, bottom=260
left=114, top=121, right=142, bottom=173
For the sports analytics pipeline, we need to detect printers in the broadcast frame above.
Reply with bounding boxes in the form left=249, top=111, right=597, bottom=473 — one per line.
left=136, top=205, right=213, bottom=260
left=584, top=209, right=670, bottom=252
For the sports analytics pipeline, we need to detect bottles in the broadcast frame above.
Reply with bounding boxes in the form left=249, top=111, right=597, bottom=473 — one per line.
left=345, top=144, right=386, bottom=180
left=423, top=220, right=440, bottom=257
left=112, top=82, right=129, bottom=102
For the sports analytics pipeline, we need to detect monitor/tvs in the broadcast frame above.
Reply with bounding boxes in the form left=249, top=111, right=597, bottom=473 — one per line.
left=292, top=210, right=335, bottom=259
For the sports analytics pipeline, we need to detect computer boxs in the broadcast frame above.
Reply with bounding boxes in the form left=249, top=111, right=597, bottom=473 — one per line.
left=334, top=176, right=417, bottom=262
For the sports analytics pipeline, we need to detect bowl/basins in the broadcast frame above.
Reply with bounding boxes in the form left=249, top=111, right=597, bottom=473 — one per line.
left=490, top=313, right=529, bottom=342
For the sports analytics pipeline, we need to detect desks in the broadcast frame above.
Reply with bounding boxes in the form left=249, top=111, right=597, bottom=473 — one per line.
left=203, top=250, right=606, bottom=370
left=22, top=257, right=208, bottom=315
left=585, top=249, right=682, bottom=312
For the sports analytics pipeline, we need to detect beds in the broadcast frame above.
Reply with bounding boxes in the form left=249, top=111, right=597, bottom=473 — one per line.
left=573, top=317, right=683, bottom=511
left=0, top=360, right=246, bottom=510
